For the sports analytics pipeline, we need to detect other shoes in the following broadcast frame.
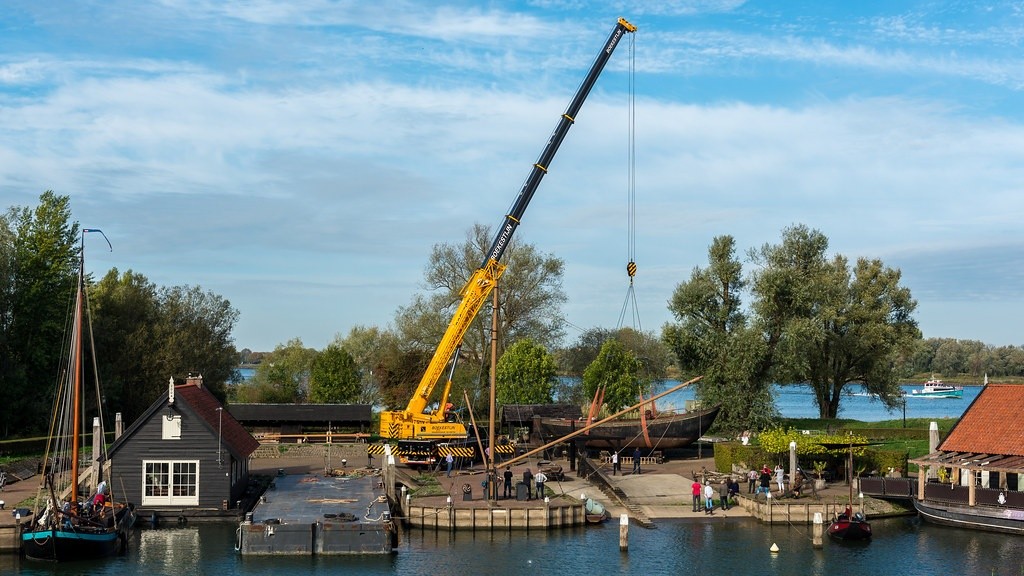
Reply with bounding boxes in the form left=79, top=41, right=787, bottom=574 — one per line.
left=447, top=475, right=451, bottom=478
left=503, top=495, right=506, bottom=498
left=102, top=514, right=105, bottom=518
left=693, top=510, right=696, bottom=512
left=698, top=510, right=700, bottom=512
left=508, top=495, right=511, bottom=498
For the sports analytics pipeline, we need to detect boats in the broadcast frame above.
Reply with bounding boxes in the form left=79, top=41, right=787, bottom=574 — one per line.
left=540, top=403, right=723, bottom=449
left=912, top=497, right=1024, bottom=537
left=912, top=370, right=963, bottom=398
left=828, top=508, right=873, bottom=541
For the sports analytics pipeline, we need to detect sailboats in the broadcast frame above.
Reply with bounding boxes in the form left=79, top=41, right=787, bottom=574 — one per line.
left=18, top=228, right=139, bottom=562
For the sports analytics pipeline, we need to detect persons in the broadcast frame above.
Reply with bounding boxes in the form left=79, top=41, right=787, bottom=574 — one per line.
left=742, top=433, right=749, bottom=446
left=577, top=448, right=587, bottom=478
left=717, top=481, right=729, bottom=511
left=704, top=481, right=714, bottom=515
left=446, top=452, right=453, bottom=478
left=632, top=446, right=642, bottom=475
left=692, top=478, right=702, bottom=512
left=887, top=465, right=903, bottom=473
left=504, top=466, right=513, bottom=498
left=747, top=464, right=803, bottom=500
left=500, top=435, right=508, bottom=459
left=485, top=445, right=490, bottom=464
left=518, top=433, right=524, bottom=444
left=535, top=469, right=548, bottom=499
left=608, top=450, right=618, bottom=476
left=523, top=468, right=533, bottom=500
left=725, top=477, right=739, bottom=501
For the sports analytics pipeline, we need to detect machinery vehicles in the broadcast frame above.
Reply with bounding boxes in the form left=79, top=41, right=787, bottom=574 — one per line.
left=370, top=15, right=638, bottom=471
